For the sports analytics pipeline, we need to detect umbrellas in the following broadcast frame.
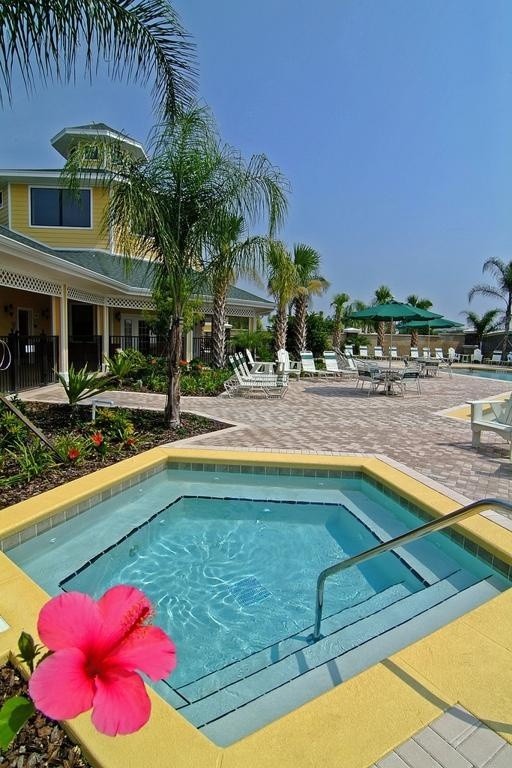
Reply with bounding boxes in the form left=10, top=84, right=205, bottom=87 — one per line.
left=347, top=296, right=444, bottom=391
left=396, top=319, right=465, bottom=374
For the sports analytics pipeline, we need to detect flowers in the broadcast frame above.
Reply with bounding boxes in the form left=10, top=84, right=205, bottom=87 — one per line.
left=0, top=583, right=177, bottom=768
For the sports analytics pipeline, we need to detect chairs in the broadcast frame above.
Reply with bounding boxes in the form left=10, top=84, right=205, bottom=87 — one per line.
left=223, top=345, right=512, bottom=400
left=464, top=398, right=512, bottom=464
